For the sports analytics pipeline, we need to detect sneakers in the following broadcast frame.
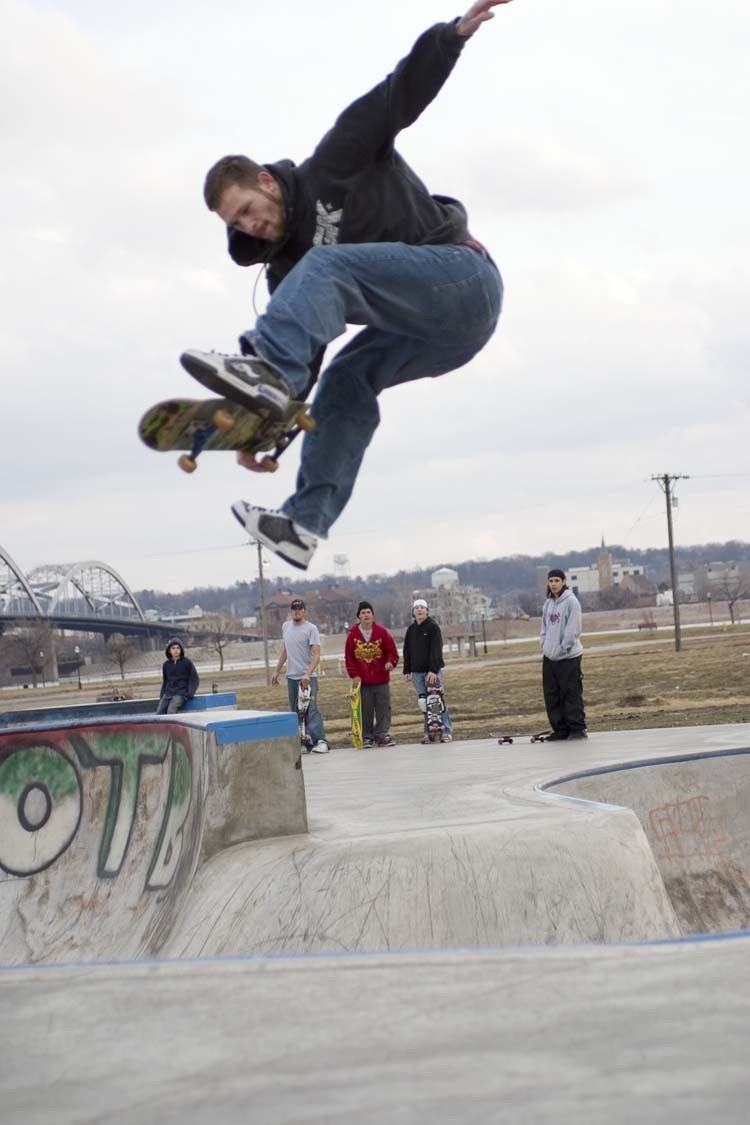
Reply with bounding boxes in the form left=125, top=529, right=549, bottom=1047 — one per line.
left=230, top=500, right=317, bottom=571
left=181, top=348, right=292, bottom=420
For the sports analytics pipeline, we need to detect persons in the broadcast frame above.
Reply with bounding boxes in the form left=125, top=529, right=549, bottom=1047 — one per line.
left=270, top=598, right=332, bottom=752
left=156, top=637, right=199, bottom=714
left=537, top=569, right=589, bottom=740
left=344, top=601, right=399, bottom=749
left=180, top=0, right=516, bottom=570
left=401, top=599, right=453, bottom=743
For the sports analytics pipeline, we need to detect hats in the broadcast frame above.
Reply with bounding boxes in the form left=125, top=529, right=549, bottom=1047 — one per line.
left=412, top=600, right=428, bottom=619
left=291, top=599, right=305, bottom=610
left=356, top=601, right=374, bottom=620
left=548, top=569, right=565, bottom=581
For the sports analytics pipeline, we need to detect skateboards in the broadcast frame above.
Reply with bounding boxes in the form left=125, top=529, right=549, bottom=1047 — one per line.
left=138, top=398, right=316, bottom=474
left=425, top=675, right=446, bottom=742
left=488, top=730, right=552, bottom=745
left=344, top=680, right=363, bottom=750
left=293, top=681, right=314, bottom=754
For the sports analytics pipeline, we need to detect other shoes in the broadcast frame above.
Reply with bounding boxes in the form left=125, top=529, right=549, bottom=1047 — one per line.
left=546, top=732, right=569, bottom=742
left=569, top=730, right=588, bottom=739
left=363, top=739, right=374, bottom=747
left=379, top=735, right=396, bottom=747
left=301, top=745, right=308, bottom=753
left=421, top=734, right=431, bottom=743
left=441, top=732, right=453, bottom=742
left=312, top=741, right=329, bottom=753
